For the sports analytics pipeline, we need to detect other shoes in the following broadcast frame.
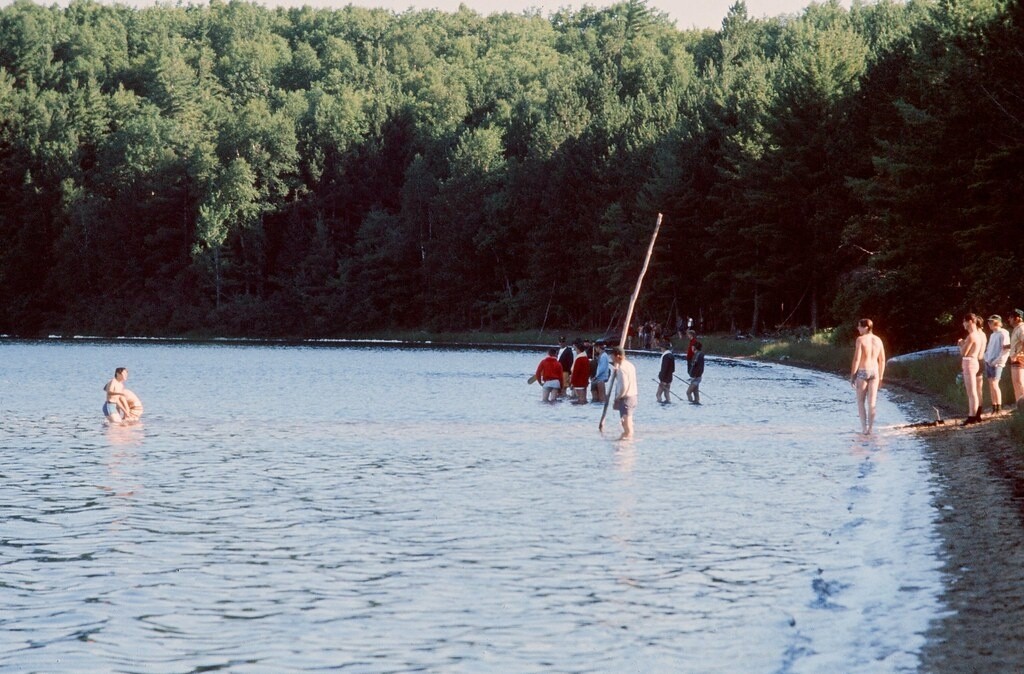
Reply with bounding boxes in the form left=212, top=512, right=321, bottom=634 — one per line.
left=959, top=416, right=977, bottom=427
left=989, top=404, right=1000, bottom=417
left=976, top=406, right=983, bottom=423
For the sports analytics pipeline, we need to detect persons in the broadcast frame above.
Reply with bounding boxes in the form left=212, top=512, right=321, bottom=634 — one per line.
left=958, top=309, right=1024, bottom=424
left=851, top=319, right=885, bottom=435
left=103, top=381, right=142, bottom=422
left=102, top=367, right=128, bottom=421
left=537, top=314, right=704, bottom=404
left=611, top=347, right=636, bottom=437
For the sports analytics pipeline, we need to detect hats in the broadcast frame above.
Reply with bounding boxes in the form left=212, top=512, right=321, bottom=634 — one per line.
left=1008, top=308, right=1023, bottom=317
left=659, top=342, right=671, bottom=350
left=987, top=315, right=1001, bottom=321
left=558, top=336, right=567, bottom=341
left=605, top=346, right=625, bottom=356
left=593, top=340, right=604, bottom=347
left=693, top=342, right=702, bottom=351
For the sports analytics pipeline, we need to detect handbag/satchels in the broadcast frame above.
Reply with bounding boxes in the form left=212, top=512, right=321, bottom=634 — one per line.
left=654, top=332, right=660, bottom=339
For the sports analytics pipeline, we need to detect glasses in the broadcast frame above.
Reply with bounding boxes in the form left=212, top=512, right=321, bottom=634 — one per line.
left=559, top=341, right=566, bottom=342
